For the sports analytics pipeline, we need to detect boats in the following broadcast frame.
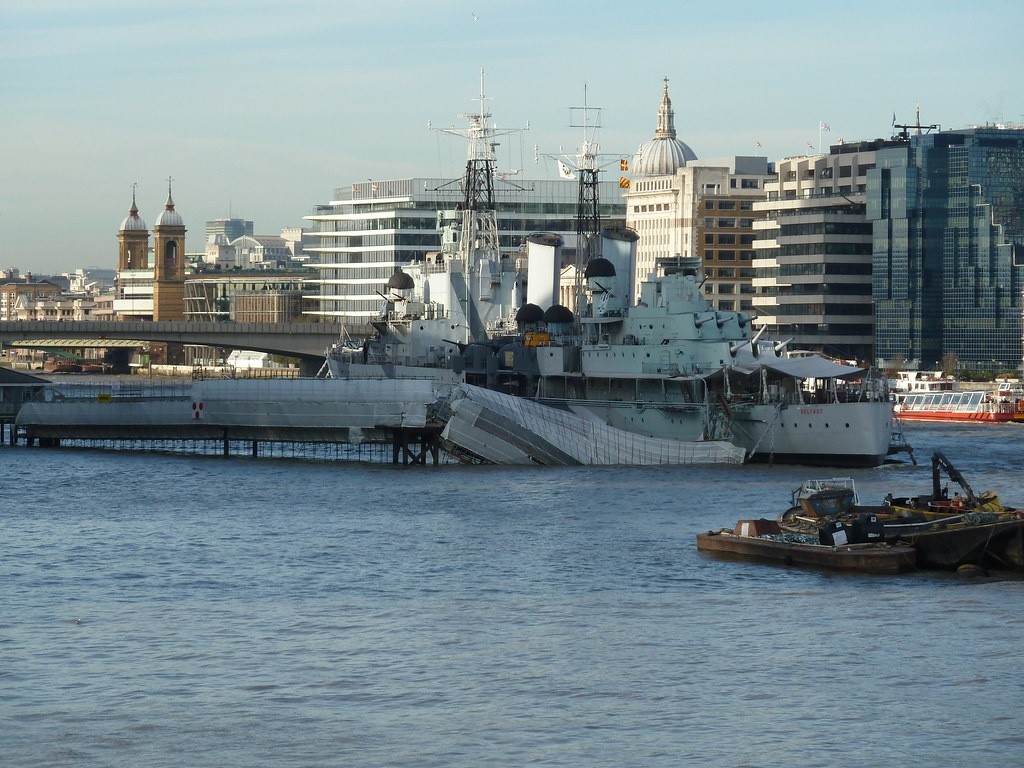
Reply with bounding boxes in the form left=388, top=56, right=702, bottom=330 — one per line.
left=697, top=452, right=1024, bottom=574
left=325, top=67, right=896, bottom=467
left=788, top=351, right=1024, bottom=423
